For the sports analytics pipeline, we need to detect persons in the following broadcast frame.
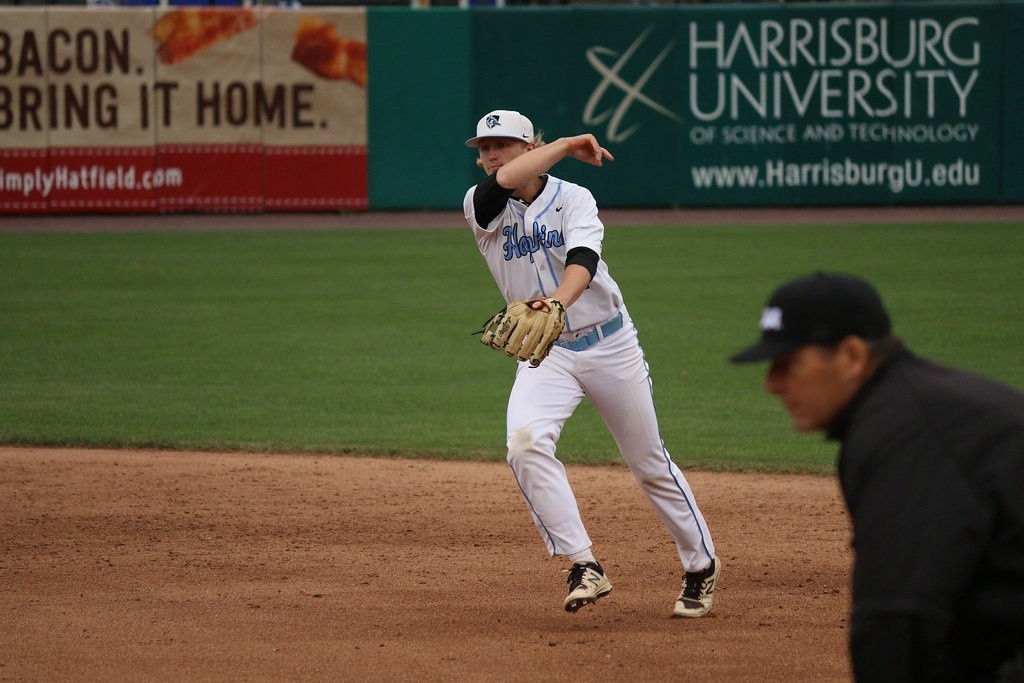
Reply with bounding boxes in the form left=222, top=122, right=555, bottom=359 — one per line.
left=727, top=268, right=1024, bottom=683
left=462, top=110, right=720, bottom=618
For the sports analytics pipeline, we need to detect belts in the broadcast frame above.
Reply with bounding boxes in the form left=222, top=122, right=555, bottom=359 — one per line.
left=553, top=311, right=623, bottom=351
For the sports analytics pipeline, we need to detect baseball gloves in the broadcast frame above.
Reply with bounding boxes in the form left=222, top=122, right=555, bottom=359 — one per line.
left=480, top=297, right=568, bottom=366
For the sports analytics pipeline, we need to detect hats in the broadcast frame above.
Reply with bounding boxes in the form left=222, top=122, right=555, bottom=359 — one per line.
left=730, top=271, right=891, bottom=364
left=465, top=110, right=534, bottom=148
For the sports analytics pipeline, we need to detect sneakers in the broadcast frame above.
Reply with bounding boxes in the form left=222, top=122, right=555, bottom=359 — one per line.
left=563, top=561, right=612, bottom=613
left=673, top=556, right=720, bottom=618
